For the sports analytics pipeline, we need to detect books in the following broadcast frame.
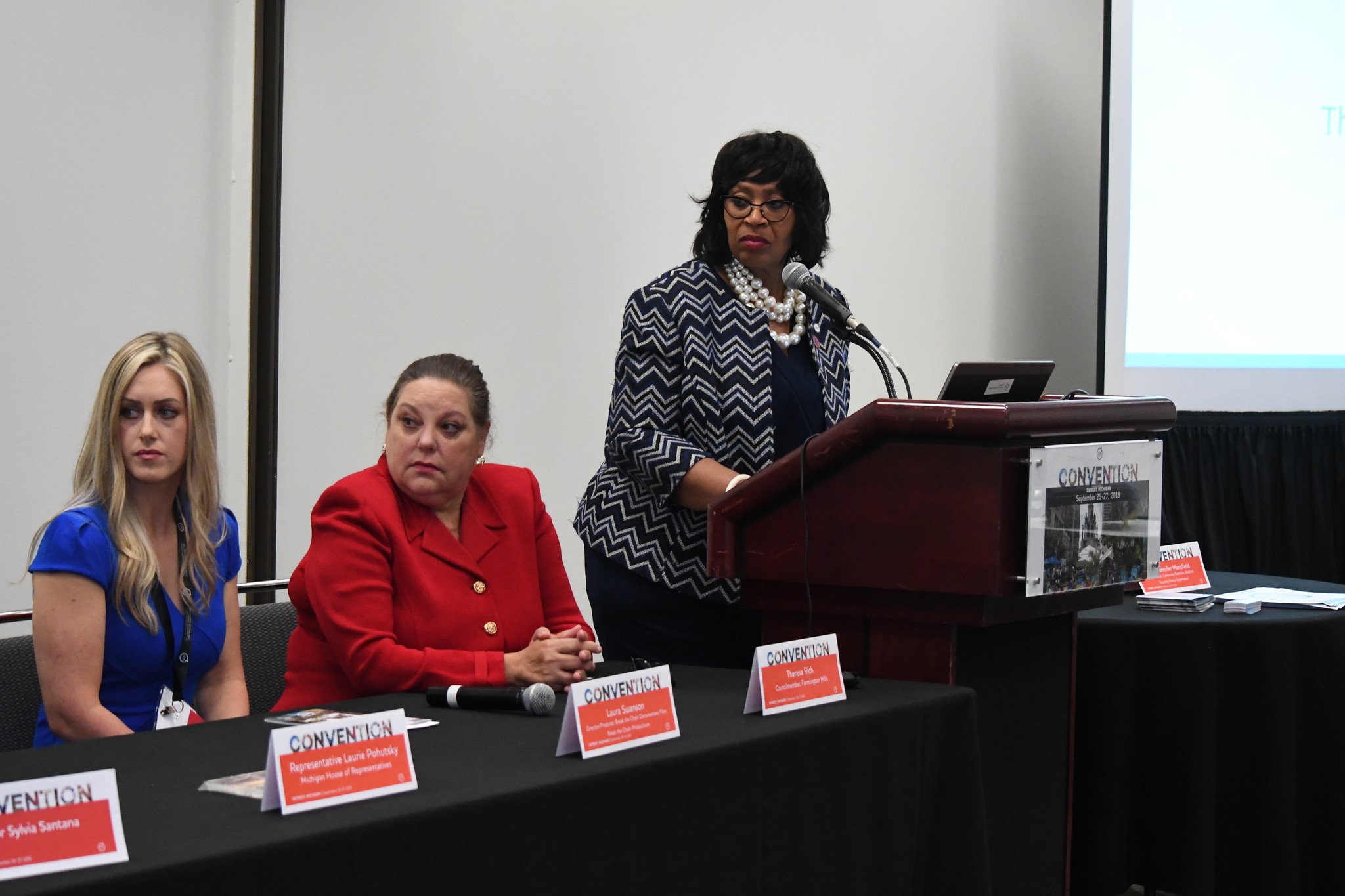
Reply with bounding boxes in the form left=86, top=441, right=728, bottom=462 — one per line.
left=1135, top=592, right=1215, bottom=613
left=1224, top=597, right=1263, bottom=616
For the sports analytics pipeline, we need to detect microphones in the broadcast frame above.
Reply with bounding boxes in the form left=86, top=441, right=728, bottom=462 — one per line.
left=781, top=262, right=875, bottom=340
left=425, top=682, right=556, bottom=715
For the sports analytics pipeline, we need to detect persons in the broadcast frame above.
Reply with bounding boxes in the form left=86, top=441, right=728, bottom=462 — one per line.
left=1043, top=556, right=1145, bottom=594
left=567, top=128, right=853, bottom=661
left=269, top=351, right=605, bottom=711
left=26, top=329, right=253, bottom=748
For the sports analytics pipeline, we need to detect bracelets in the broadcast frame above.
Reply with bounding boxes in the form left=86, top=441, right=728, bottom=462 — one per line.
left=724, top=473, right=750, bottom=493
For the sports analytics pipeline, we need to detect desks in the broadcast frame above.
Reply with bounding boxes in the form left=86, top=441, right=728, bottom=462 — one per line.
left=0, top=657, right=976, bottom=896
left=1067, top=570, right=1345, bottom=896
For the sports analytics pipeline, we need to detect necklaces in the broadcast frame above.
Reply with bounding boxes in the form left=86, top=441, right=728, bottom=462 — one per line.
left=722, top=257, right=810, bottom=349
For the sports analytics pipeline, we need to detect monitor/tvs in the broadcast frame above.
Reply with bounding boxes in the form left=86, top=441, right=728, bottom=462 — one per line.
left=936, top=360, right=1056, bottom=402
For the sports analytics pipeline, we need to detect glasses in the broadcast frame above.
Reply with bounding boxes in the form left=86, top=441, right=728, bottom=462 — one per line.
left=631, top=656, right=677, bottom=687
left=718, top=193, right=794, bottom=223
